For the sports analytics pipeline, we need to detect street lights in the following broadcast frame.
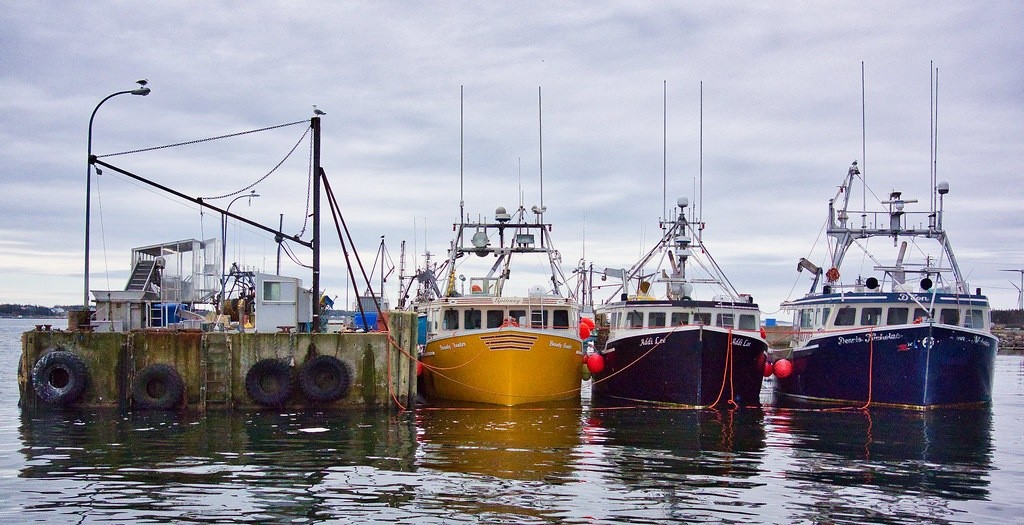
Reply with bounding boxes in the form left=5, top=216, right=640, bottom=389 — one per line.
left=84, top=87, right=152, bottom=308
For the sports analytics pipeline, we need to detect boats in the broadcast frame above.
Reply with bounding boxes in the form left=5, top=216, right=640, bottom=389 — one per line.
left=582, top=82, right=769, bottom=407
left=415, top=60, right=585, bottom=408
left=768, top=57, right=998, bottom=415
